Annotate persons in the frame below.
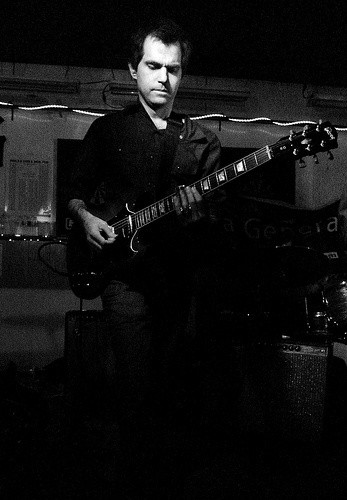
[52,12,232,472]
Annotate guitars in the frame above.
[66,118,339,300]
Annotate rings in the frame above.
[179,203,193,218]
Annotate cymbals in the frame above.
[263,242,330,288]
